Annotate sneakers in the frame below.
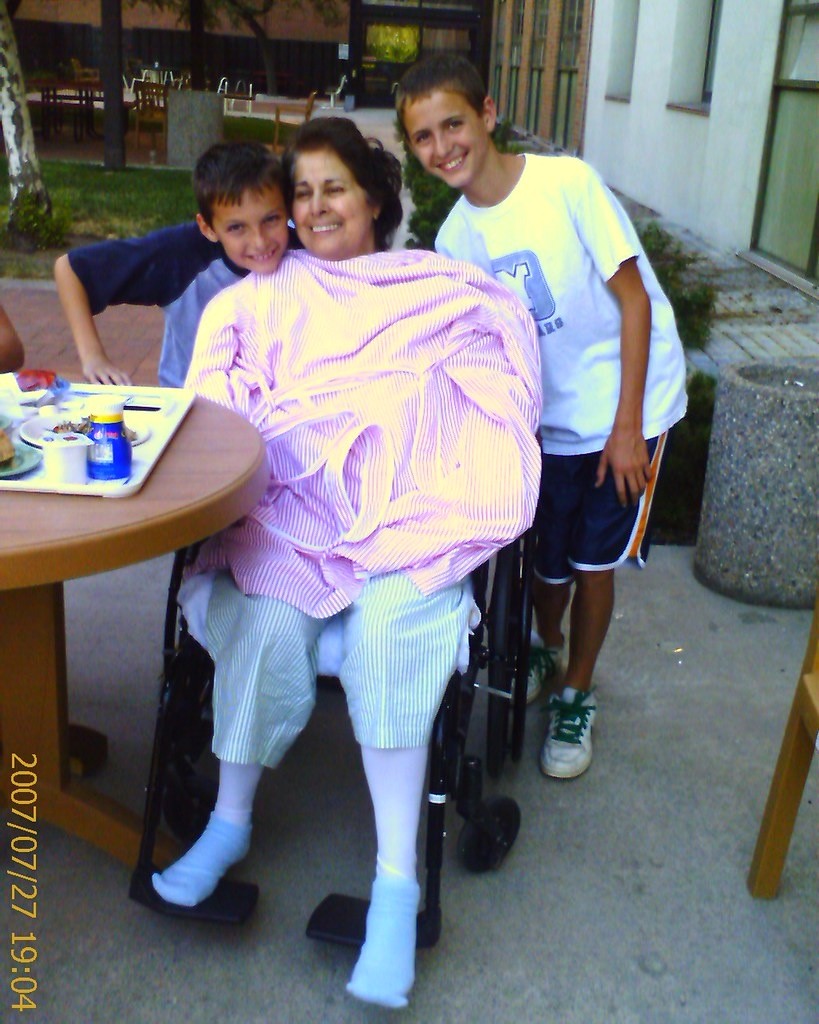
[511,628,565,703]
[541,685,599,779]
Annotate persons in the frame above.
[0,303,25,373]
[52,139,308,389]
[399,52,690,781]
[152,116,545,1008]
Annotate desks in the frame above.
[0,382,269,873]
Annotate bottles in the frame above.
[86,396,132,481]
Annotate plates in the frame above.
[19,410,152,457]
[14,374,71,395]
[0,444,43,480]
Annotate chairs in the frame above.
[271,88,319,154]
[323,74,348,109]
[24,68,261,158]
